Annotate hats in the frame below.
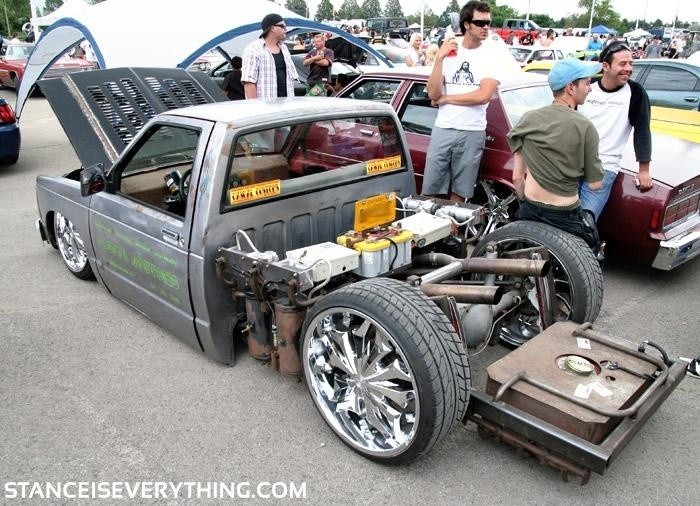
[548,56,603,92]
[258,13,283,38]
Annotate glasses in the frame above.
[466,19,491,28]
[274,24,287,29]
[603,41,627,62]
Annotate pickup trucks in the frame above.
[490,19,550,41]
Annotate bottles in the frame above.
[445,24,458,58]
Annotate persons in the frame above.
[402,28,700,67]
[222,13,365,148]
[71,43,85,60]
[506,60,600,276]
[575,41,654,221]
[420,0,498,233]
[450,61,473,84]
[88,44,95,63]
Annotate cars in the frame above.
[0,43,98,90]
[524,58,700,142]
[510,44,563,67]
[275,71,700,270]
[322,18,464,67]
[34,68,700,484]
[0,96,20,166]
